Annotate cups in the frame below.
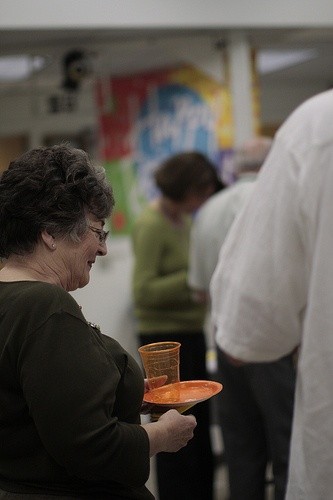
[137,342,181,403]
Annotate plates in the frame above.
[142,378,223,406]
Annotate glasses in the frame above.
[88,225,109,246]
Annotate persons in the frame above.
[211,89,333,500]
[0,144,197,500]
[187,137,297,500]
[132,150,228,500]
